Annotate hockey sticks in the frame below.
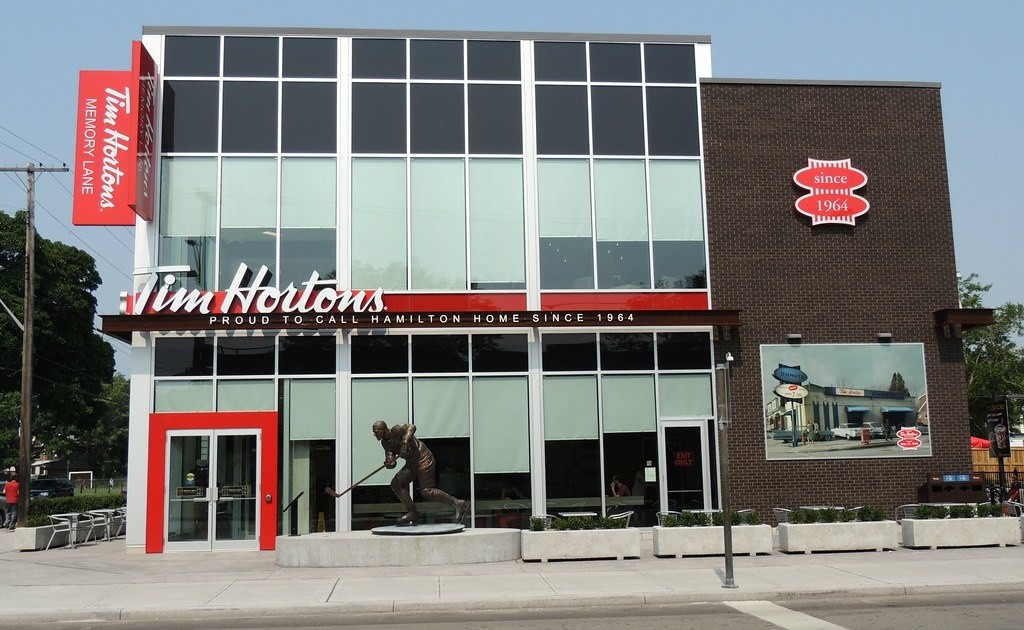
[325,448,412,498]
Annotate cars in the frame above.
[0,481,9,528]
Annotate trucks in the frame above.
[771,429,834,443]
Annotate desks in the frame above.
[558,511,598,530]
[923,501,978,519]
[800,506,845,522]
[683,509,724,526]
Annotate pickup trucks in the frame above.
[830,423,861,440]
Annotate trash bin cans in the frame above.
[917,473,957,503]
[954,473,986,503]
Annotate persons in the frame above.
[605,466,652,527]
[804,417,895,449]
[0,471,116,532]
[372,420,472,526]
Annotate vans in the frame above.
[862,421,887,439]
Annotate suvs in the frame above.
[29,476,75,501]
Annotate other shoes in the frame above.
[3,523,16,530]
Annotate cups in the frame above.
[994,424,1007,449]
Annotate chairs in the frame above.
[92,509,126,541]
[608,510,634,528]
[894,503,924,522]
[76,512,112,545]
[503,502,529,515]
[979,502,992,517]
[529,514,560,531]
[773,507,793,524]
[737,509,753,523]
[652,499,678,510]
[848,506,864,522]
[1002,500,1024,517]
[656,511,684,527]
[45,512,94,550]
